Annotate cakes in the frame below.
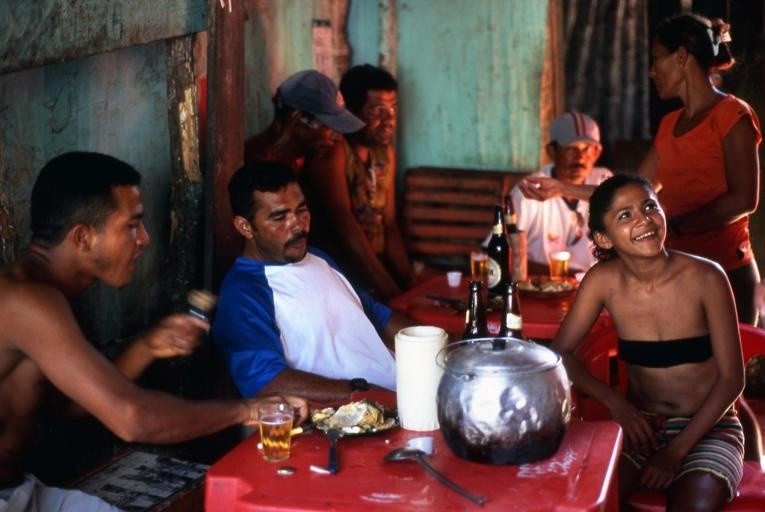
[328,404,385,430]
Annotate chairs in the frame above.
[620,319,765,512]
[402,167,505,261]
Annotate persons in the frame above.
[219,160,430,413]
[518,14,762,330]
[481,110,613,272]
[243,69,364,259]
[338,63,423,308]
[551,174,746,511]
[2,151,308,510]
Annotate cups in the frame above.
[471,250,489,312]
[258,403,293,461]
[551,253,568,281]
[448,272,462,287]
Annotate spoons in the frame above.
[383,448,486,503]
[325,428,345,473]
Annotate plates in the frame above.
[309,406,400,435]
[515,280,576,298]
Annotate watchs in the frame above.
[669,215,684,236]
[244,397,260,428]
[348,378,369,392]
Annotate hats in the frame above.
[273,70,367,135]
[548,110,602,151]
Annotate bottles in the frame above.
[488,209,508,304]
[464,282,489,340]
[497,284,522,340]
[504,208,526,281]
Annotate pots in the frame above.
[437,338,571,464]
